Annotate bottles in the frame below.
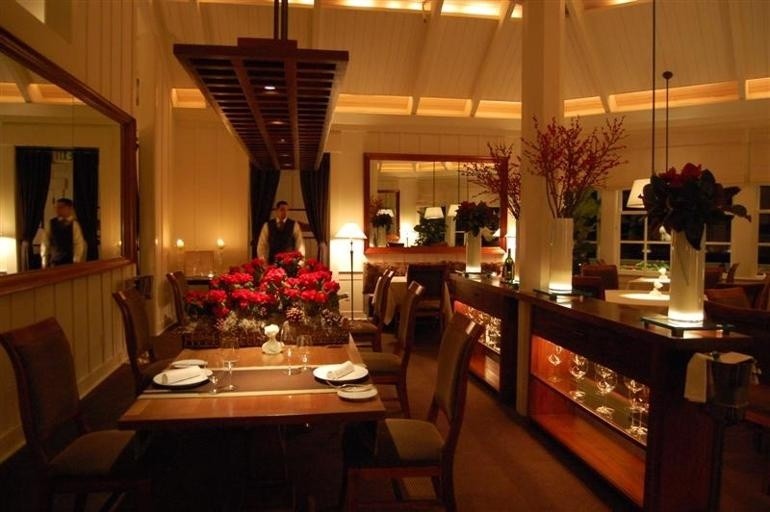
[504,248,513,281]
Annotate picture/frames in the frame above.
[184,248,214,278]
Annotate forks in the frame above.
[325,380,368,388]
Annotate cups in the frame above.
[623,375,644,435]
[593,365,617,415]
[297,334,313,374]
[204,354,225,395]
[568,352,589,398]
[281,326,301,376]
[629,389,649,437]
[221,337,240,392]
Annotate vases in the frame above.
[547,216,576,294]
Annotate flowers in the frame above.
[522,113,631,211]
[184,250,353,347]
[455,142,524,218]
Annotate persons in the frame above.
[257,201,305,269]
[40,198,85,268]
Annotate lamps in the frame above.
[627,3,655,208]
[447,162,462,218]
[425,161,444,223]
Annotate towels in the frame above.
[160,365,200,384]
[327,360,353,379]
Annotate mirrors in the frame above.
[361,151,508,254]
[0,25,139,291]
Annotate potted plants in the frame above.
[372,213,390,248]
[451,203,498,273]
[644,161,753,322]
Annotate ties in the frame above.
[279,221,283,228]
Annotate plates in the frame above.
[338,385,378,400]
[173,359,204,368]
[313,363,369,382]
[153,369,213,387]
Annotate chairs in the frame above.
[113,289,174,397]
[703,287,750,308]
[573,274,608,304]
[700,294,767,383]
[718,261,743,286]
[2,315,145,510]
[339,312,485,511]
[348,269,396,351]
[406,263,447,340]
[166,269,200,350]
[577,261,619,288]
[361,282,429,416]
[699,266,724,283]
